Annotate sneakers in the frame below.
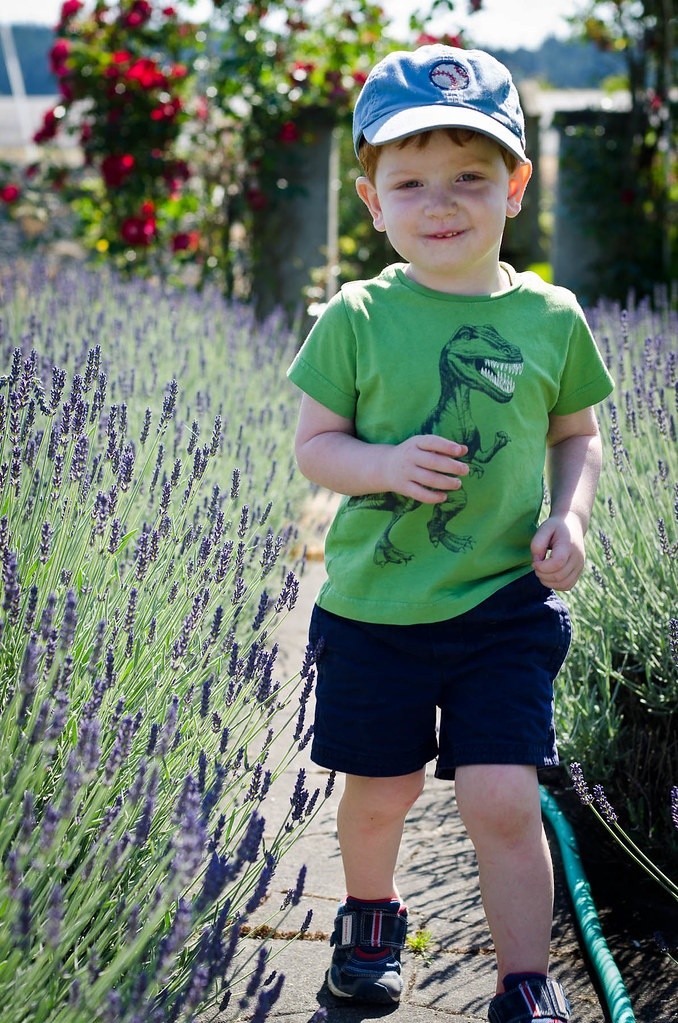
[327,893,408,1005]
[488,973,571,1023]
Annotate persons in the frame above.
[287,42,614,1023]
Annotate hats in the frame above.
[351,43,526,163]
